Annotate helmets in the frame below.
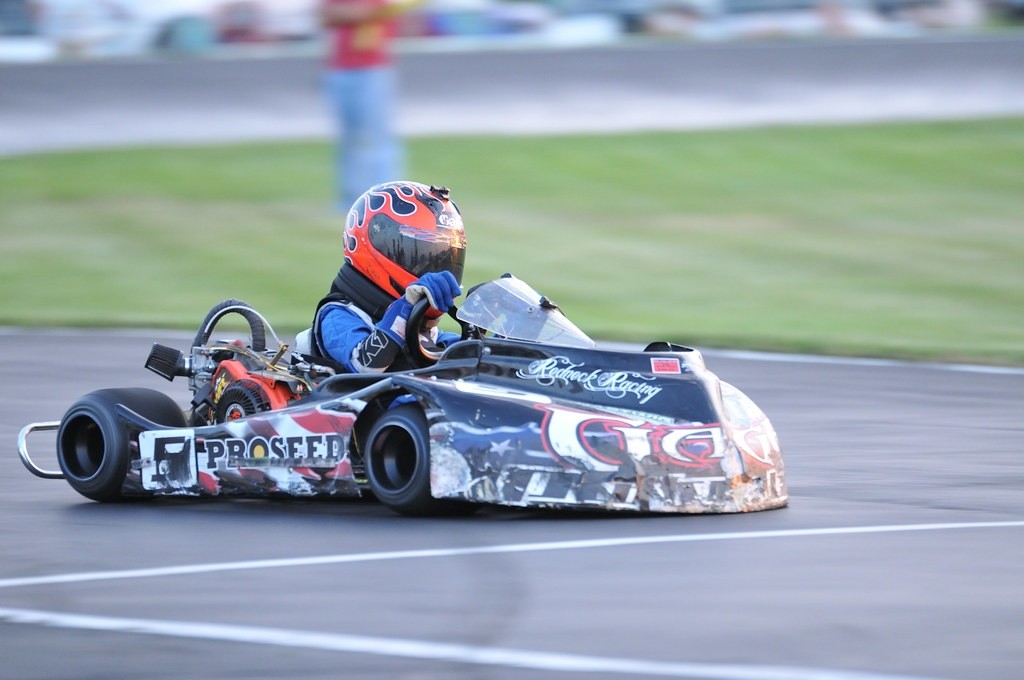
[343,180,467,318]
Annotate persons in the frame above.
[325,2,417,211]
[312,181,474,377]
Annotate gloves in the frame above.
[375,270,461,349]
